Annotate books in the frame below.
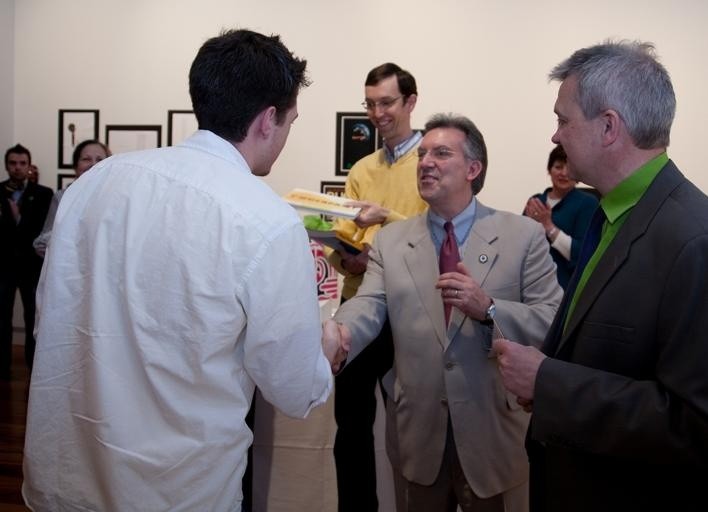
[283,187,363,221]
[308,228,364,256]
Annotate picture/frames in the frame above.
[57,109,199,190]
[320,111,384,222]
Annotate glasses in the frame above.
[361,95,405,109]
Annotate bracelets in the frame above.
[522,146,600,292]
[547,226,557,238]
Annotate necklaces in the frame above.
[548,193,559,204]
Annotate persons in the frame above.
[32,138,111,256]
[29,164,39,183]
[492,36,708,511]
[332,61,429,510]
[320,111,565,512]
[0,142,53,373]
[19,24,343,512]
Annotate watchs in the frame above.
[485,298,496,319]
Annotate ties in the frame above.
[540,204,606,356]
[440,222,460,330]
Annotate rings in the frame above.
[534,212,538,215]
[455,290,459,298]
